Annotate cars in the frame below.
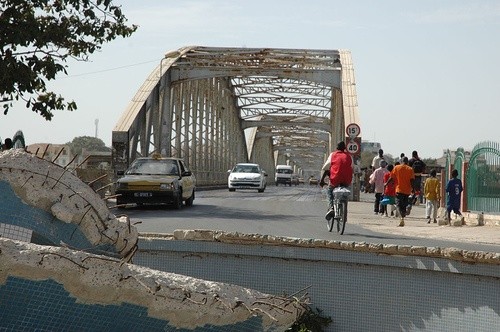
[227,163,268,193]
[114,148,197,209]
[291,171,318,186]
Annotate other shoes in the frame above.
[374,209,465,227]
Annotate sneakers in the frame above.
[324,209,333,220]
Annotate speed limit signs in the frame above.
[345,139,361,155]
[346,123,360,136]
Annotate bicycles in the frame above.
[324,181,352,235]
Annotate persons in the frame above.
[444,169,466,225]
[383,157,416,227]
[423,170,441,223]
[364,149,426,218]
[319,140,353,220]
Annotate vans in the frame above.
[274,165,293,187]
